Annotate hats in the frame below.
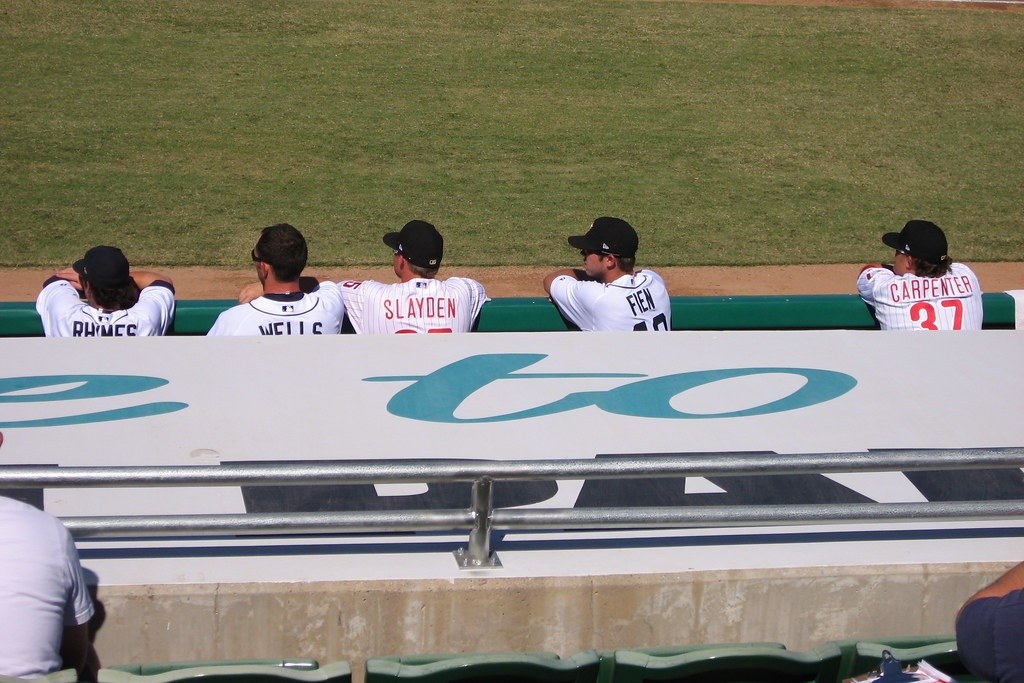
[382,220,444,269]
[567,216,639,257]
[72,245,129,283]
[882,220,947,263]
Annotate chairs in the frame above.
[3,637,983,683]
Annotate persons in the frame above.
[856,220,984,331]
[542,217,671,331]
[954,563,1024,683]
[208,224,344,334]
[0,491,97,681]
[338,221,492,333]
[36,246,177,337]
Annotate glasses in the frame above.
[251,248,272,265]
